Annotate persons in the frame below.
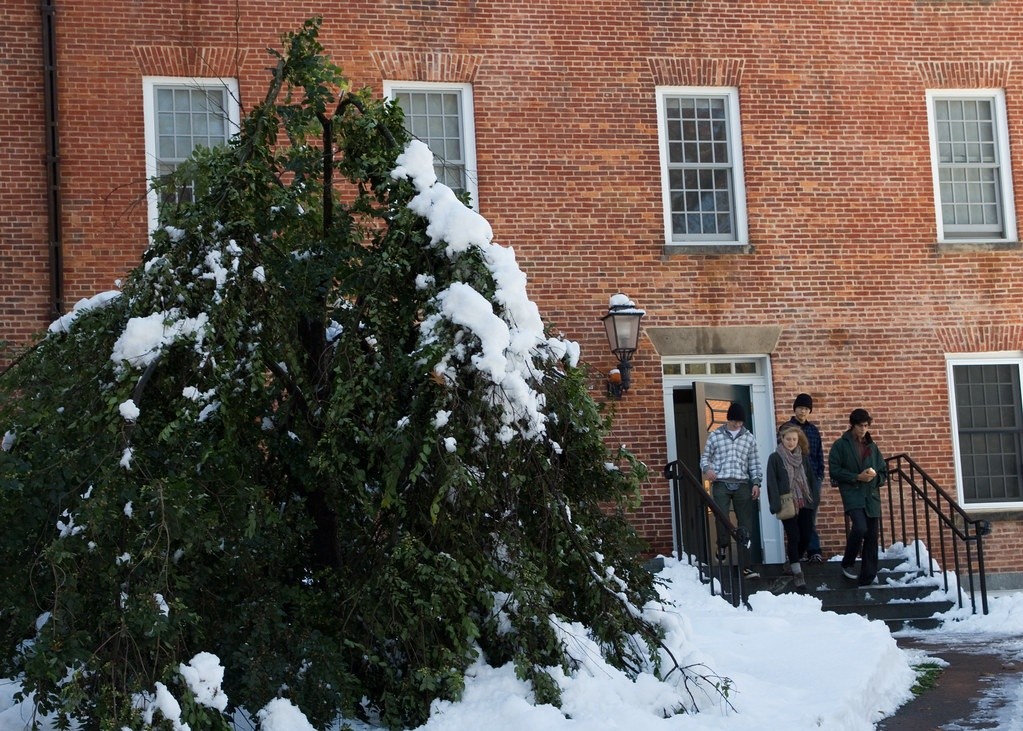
[777,393,825,563]
[768,426,814,587]
[700,403,763,579]
[828,408,888,586]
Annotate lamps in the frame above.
[599,293,645,399]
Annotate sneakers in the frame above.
[744,567,760,579]
[715,546,727,563]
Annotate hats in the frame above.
[727,403,745,421]
[793,394,812,413]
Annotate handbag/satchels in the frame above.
[775,493,795,519]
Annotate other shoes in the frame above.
[783,560,792,574]
[809,553,823,564]
[841,565,858,579]
[858,582,886,589]
[793,572,808,592]
[799,552,808,561]
